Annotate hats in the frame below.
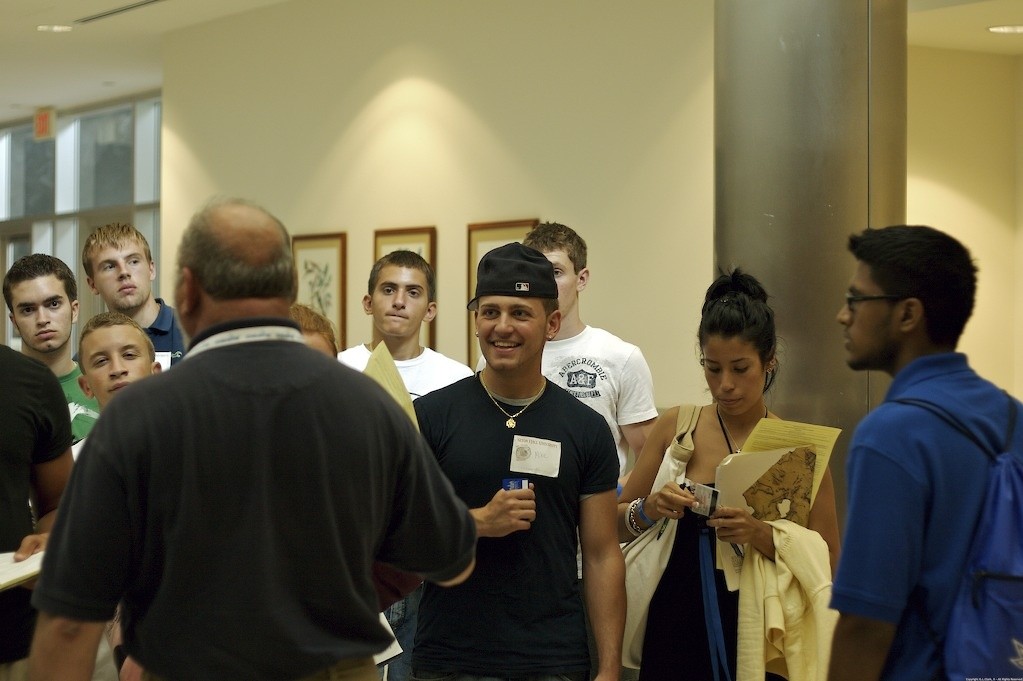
[465,242,559,313]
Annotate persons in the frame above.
[288,302,337,359]
[412,241,626,681]
[475,221,659,681]
[335,250,473,681]
[826,224,1023,681]
[613,270,839,681]
[23,204,478,681]
[0,224,185,681]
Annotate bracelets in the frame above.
[625,496,655,537]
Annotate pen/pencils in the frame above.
[657,482,686,540]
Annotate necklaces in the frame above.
[718,404,767,453]
[481,368,546,429]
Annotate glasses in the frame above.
[843,292,903,309]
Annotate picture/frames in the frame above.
[466,219,540,371]
[373,226,436,354]
[289,233,348,352]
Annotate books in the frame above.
[0,551,44,592]
